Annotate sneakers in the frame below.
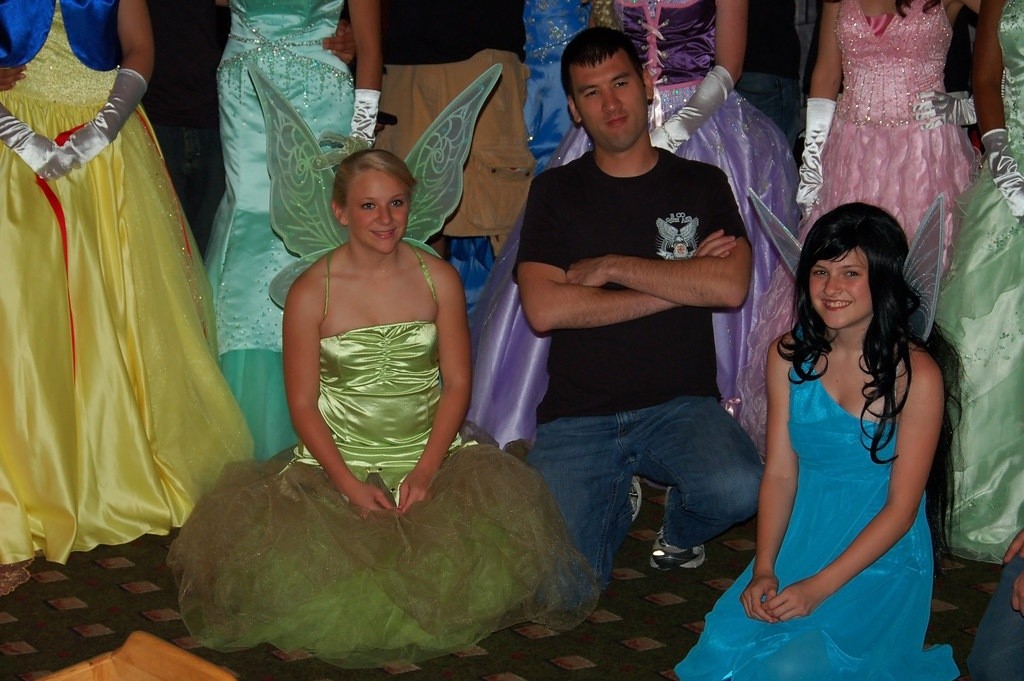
[629,473,642,522]
[649,485,706,570]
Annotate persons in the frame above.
[735,0,979,473]
[464,1,800,453]
[0,0,252,564]
[967,528,1024,680]
[170,145,597,669]
[908,0,1024,562]
[201,1,594,457]
[665,200,964,681]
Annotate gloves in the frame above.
[0,68,148,183]
[796,98,837,220]
[649,64,735,156]
[981,127,1024,226]
[349,89,382,150]
[912,90,978,132]
